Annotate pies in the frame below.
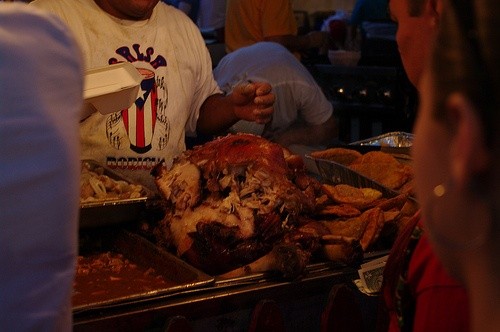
[317,183,408,252]
[312,148,406,189]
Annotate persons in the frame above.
[390,0,500,332]
[330,0,401,69]
[26,0,275,186]
[177,0,331,60]
[0,0,87,332]
[213,42,337,159]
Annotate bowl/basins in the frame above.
[328,50,362,66]
[82,62,146,116]
